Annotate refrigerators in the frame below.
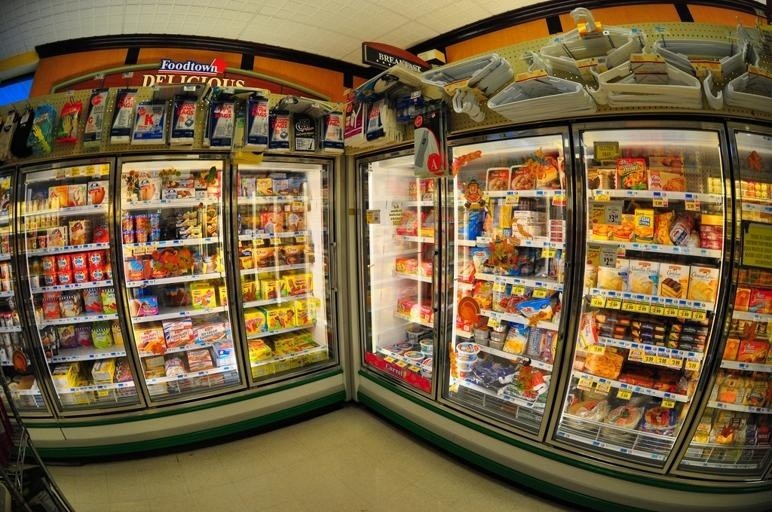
[0,149,347,462]
[345,133,771,512]
